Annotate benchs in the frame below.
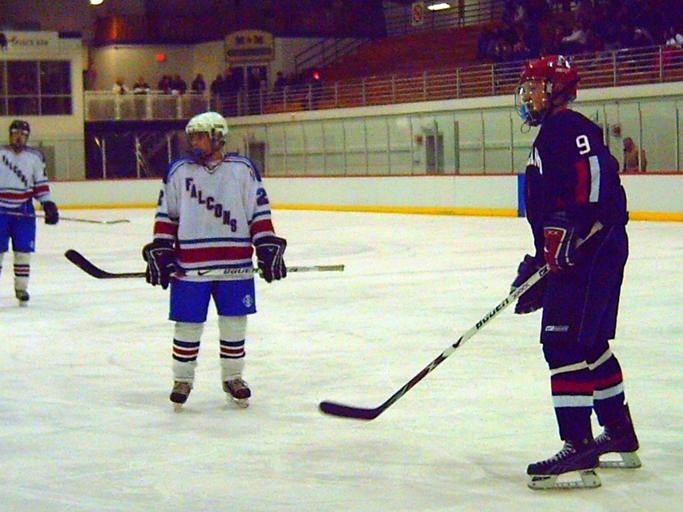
[263,0,683,112]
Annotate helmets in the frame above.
[516,55,579,127]
[185,112,228,158]
[9,120,30,137]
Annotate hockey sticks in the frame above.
[63,249,344,279]
[319,224,603,421]
[0,210,130,225]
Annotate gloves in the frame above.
[142,244,183,290]
[513,255,548,315]
[254,235,288,283]
[542,217,580,273]
[43,201,59,225]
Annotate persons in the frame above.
[0,119,59,302]
[509,52,641,477]
[113,65,322,117]
[622,137,646,172]
[475,1,683,93]
[144,110,287,402]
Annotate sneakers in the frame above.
[170,381,190,404]
[595,410,639,455]
[16,291,29,301]
[527,434,600,476]
[223,379,251,399]
